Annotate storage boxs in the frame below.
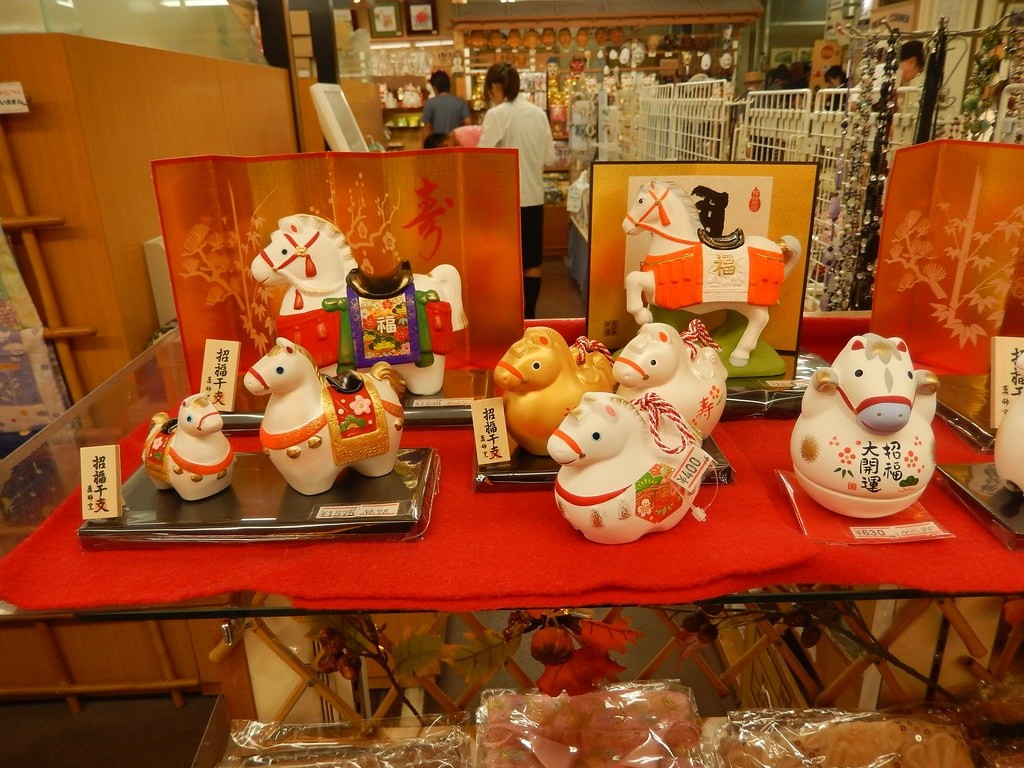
[150,332,192,409]
[143,232,179,329]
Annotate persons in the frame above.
[424,125,481,149]
[849,40,929,112]
[475,62,556,320]
[825,66,847,111]
[420,71,472,150]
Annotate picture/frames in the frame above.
[405,0,437,36]
[369,0,402,38]
